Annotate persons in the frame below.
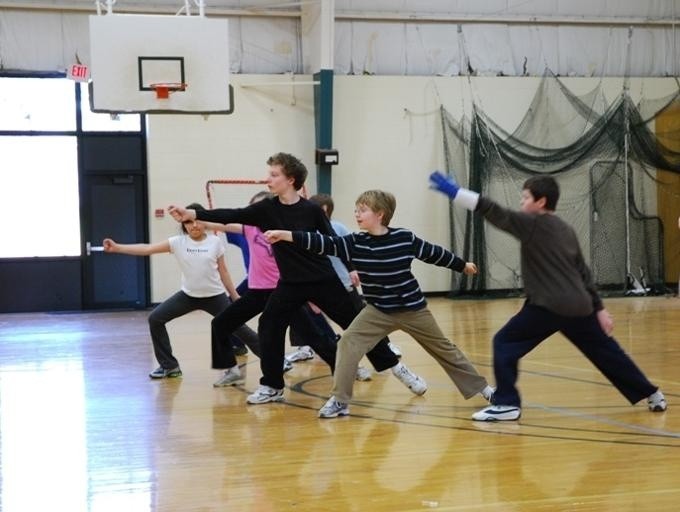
[264,188,497,419]
[168,152,428,406]
[427,169,668,422]
[102,204,293,378]
[287,193,407,362]
[193,190,373,386]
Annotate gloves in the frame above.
[427,170,479,212]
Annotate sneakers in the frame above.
[213,373,245,387]
[388,343,402,359]
[150,366,182,378]
[648,393,667,411]
[246,385,285,404]
[391,365,428,396]
[318,396,349,418]
[471,405,521,421]
[283,345,315,373]
[233,345,248,356]
[356,364,372,381]
